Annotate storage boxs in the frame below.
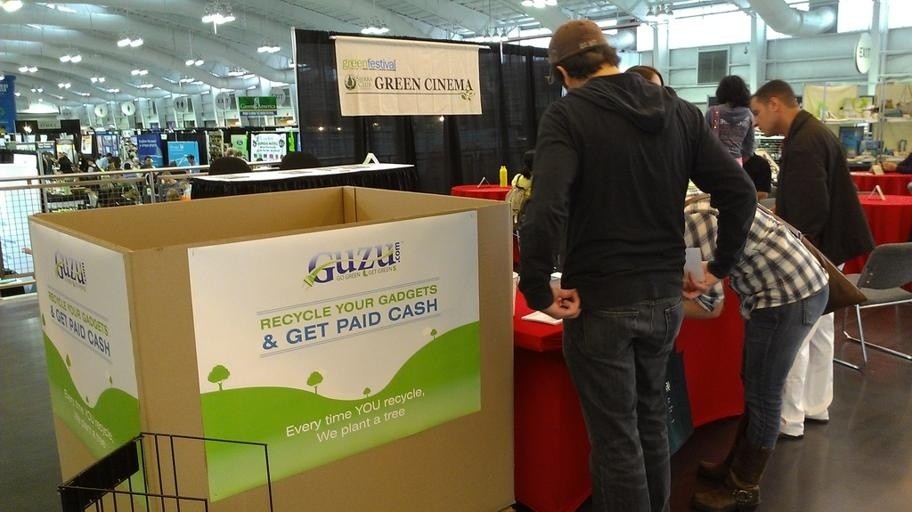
[24,182,530,511]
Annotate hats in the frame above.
[546,18,608,85]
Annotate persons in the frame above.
[881,152,912,173]
[40,149,200,194]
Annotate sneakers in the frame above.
[692,459,762,510]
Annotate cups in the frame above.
[512,270,521,319]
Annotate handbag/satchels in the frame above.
[801,233,868,317]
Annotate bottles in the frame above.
[499,165,508,188]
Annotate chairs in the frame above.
[831,238,912,378]
[758,198,777,210]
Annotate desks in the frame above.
[443,176,525,265]
[505,264,769,512]
[187,157,411,188]
[848,169,911,197]
[840,192,912,302]
